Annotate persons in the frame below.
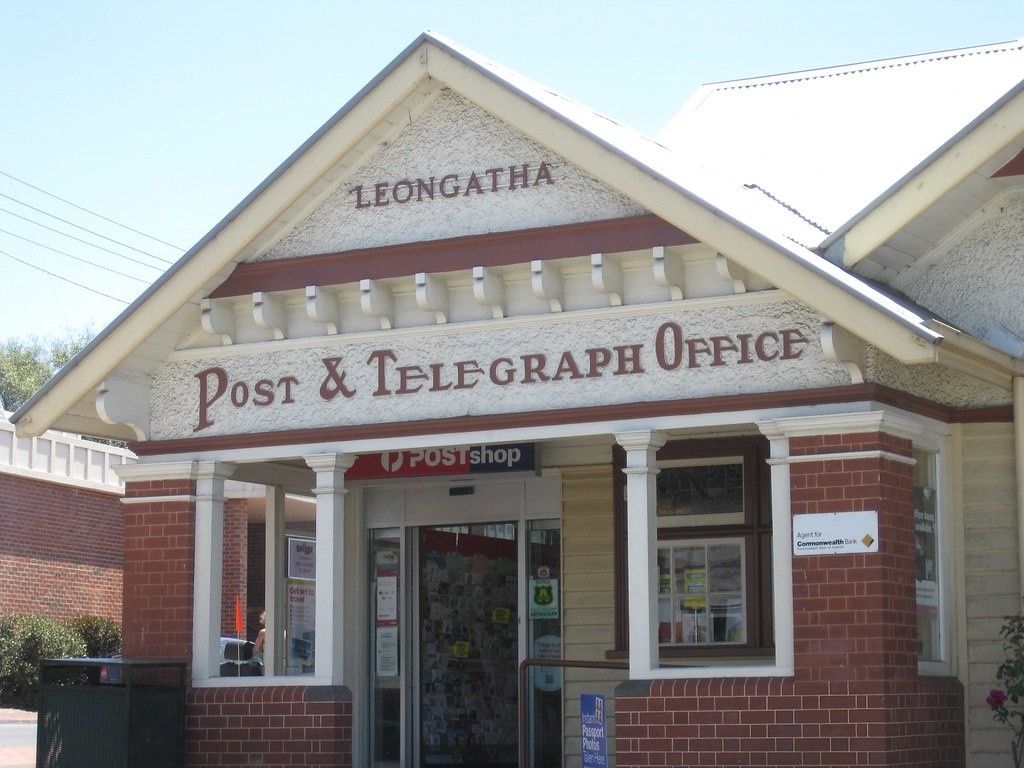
[254,612,287,676]
